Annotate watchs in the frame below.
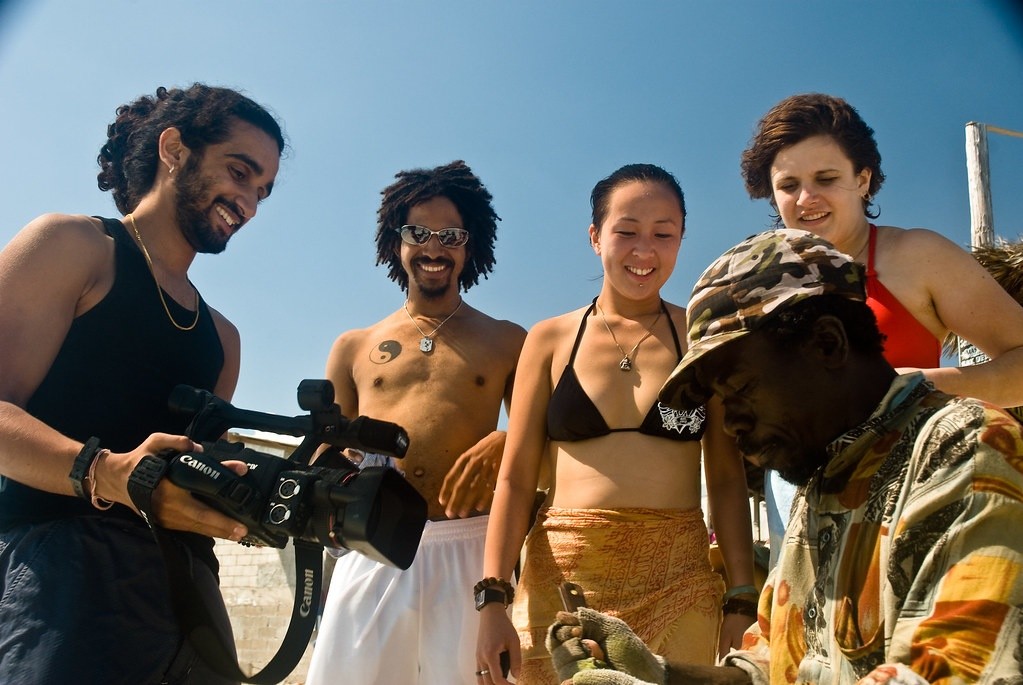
[475,587,509,611]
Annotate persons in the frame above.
[474,164,759,685]
[544,226,1023,685]
[739,91,1023,577]
[311,156,555,685]
[0,78,284,685]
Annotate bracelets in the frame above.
[68,435,115,511]
[721,597,758,620]
[473,576,515,604]
[721,584,760,604]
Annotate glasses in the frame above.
[394,224,475,249]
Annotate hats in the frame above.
[658,227,866,411]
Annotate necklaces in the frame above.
[123,213,200,331]
[594,299,664,371]
[403,293,464,353]
[852,236,870,260]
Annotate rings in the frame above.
[481,668,490,675]
[475,669,482,675]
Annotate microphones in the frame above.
[335,416,410,460]
